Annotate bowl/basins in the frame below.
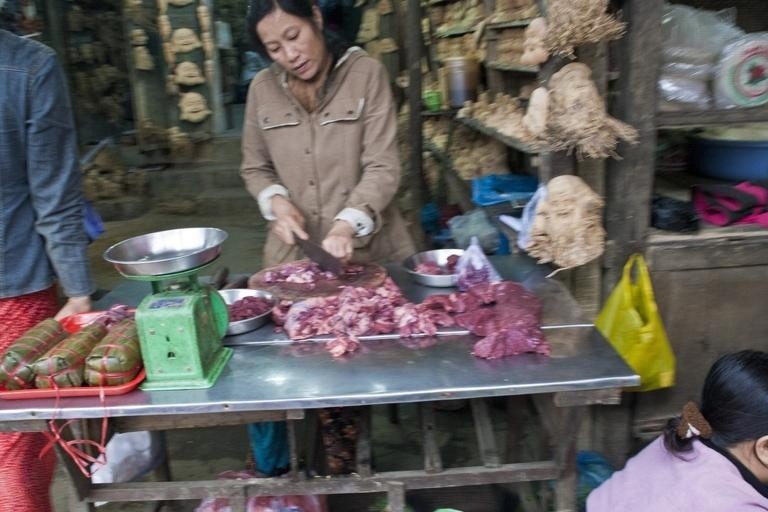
[690,132,768,182]
[216,288,278,336]
[400,248,466,287]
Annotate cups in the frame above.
[437,55,481,109]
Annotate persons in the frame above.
[578,348,767,512]
[237,0,417,275]
[0,27,96,510]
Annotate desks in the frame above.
[0,250,644,510]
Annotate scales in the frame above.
[102,228,235,393]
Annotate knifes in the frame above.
[293,233,346,279]
